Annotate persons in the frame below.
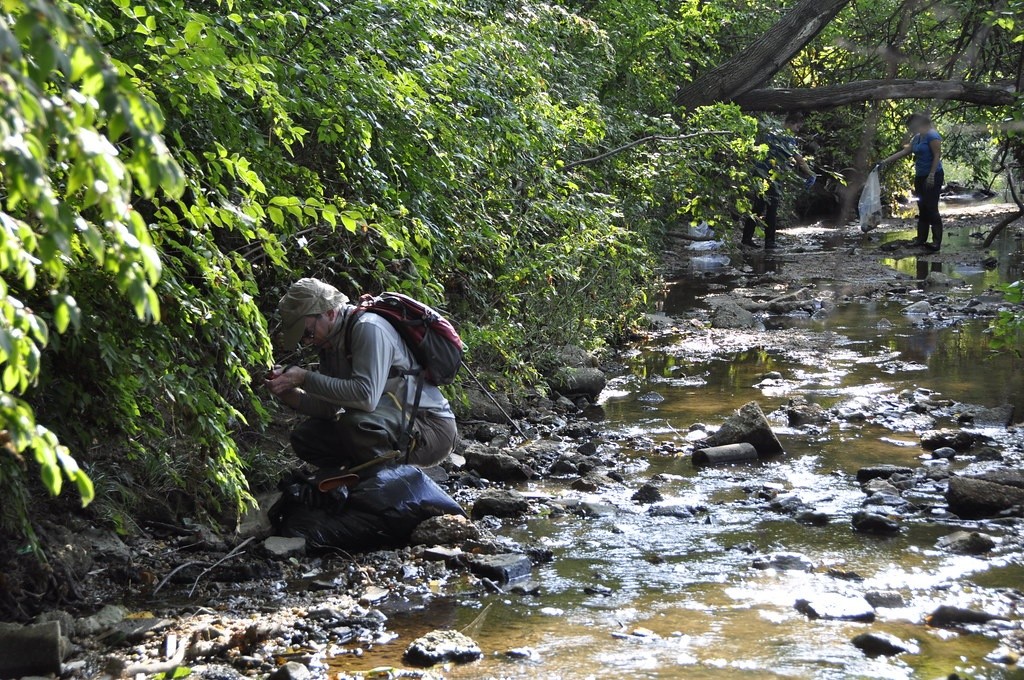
[264,277,458,480]
[741,111,816,249]
[877,113,944,252]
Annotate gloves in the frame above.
[925,172,934,189]
[805,177,815,189]
[877,159,890,171]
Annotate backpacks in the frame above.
[344,290,469,386]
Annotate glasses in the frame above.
[304,316,318,339]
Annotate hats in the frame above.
[284,317,303,349]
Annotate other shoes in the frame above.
[925,242,941,252]
[907,238,924,245]
[765,243,778,249]
[742,238,762,248]
